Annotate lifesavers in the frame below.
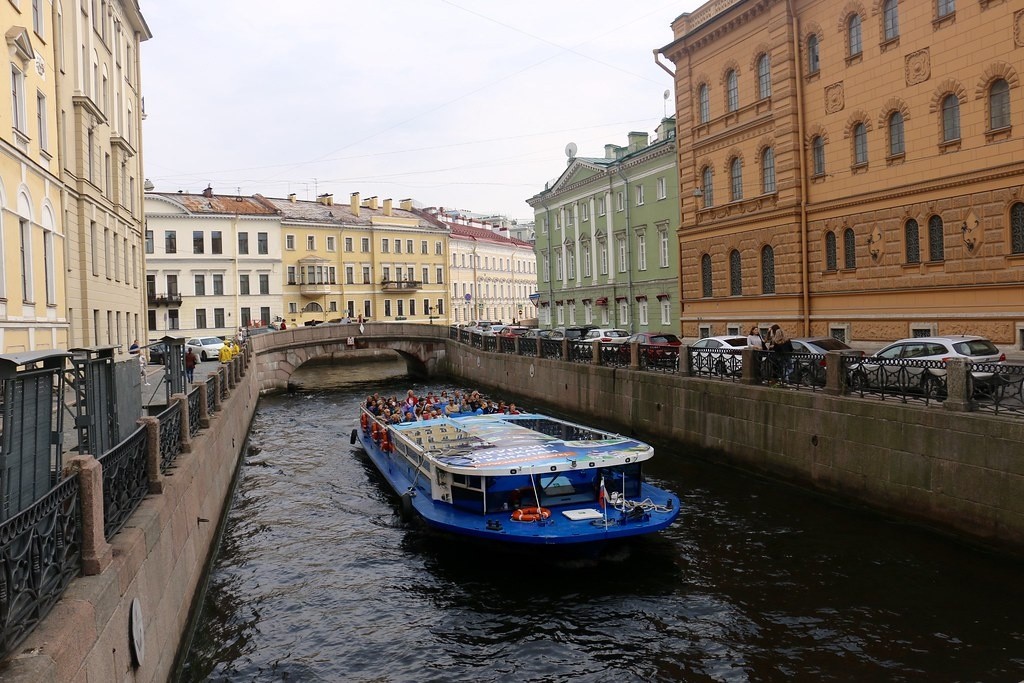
[400,494,412,521]
[361,413,367,433]
[380,428,387,451]
[513,507,551,521]
[371,422,377,443]
[350,429,357,445]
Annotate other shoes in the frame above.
[191,381,193,383]
[145,383,151,386]
[188,379,190,382]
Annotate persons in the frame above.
[911,347,919,354]
[748,326,764,381]
[186,347,195,384]
[219,340,232,364]
[138,350,152,386]
[129,340,140,353]
[765,324,780,385]
[231,341,239,355]
[234,313,363,344]
[774,330,795,390]
[484,320,557,336]
[367,390,522,424]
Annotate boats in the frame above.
[351,396,683,547]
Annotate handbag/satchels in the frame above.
[762,341,767,350]
[774,340,794,353]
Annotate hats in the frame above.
[223,340,229,344]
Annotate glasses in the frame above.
[397,413,399,414]
[449,400,454,401]
[424,413,428,414]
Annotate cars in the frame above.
[573,328,633,353]
[846,334,1012,401]
[150,344,170,365]
[763,336,866,385]
[521,329,552,339]
[452,319,505,337]
[185,337,234,362]
[544,326,590,346]
[675,335,750,375]
[494,326,530,339]
[619,331,682,359]
